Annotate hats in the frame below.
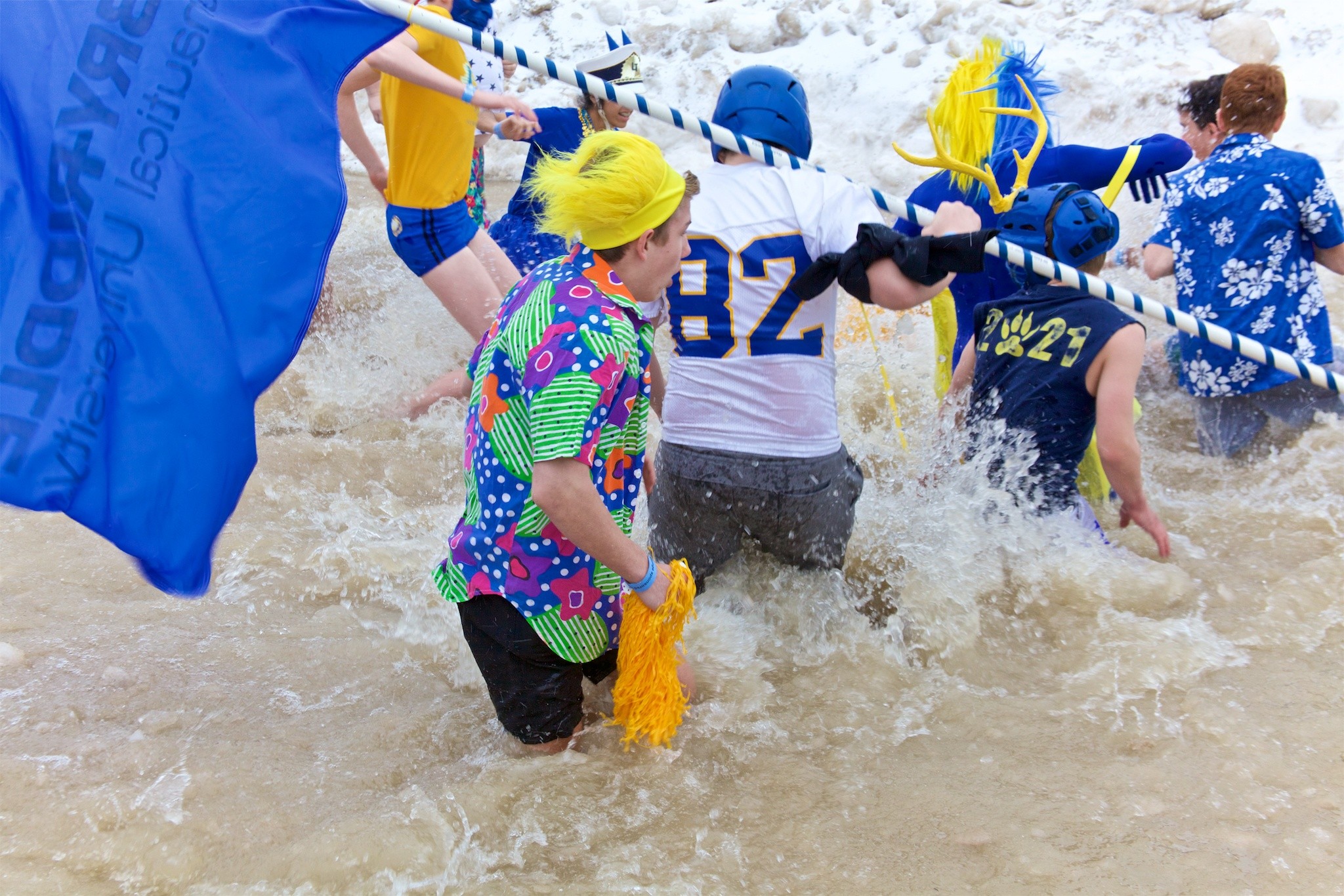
[996,181,1119,284]
[576,43,647,95]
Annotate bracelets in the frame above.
[625,556,657,592]
[460,84,475,103]
[494,122,508,141]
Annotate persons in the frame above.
[365,9,518,233]
[1178,74,1235,163]
[427,128,692,748]
[1138,61,1344,457]
[627,63,981,596]
[941,181,1170,558]
[475,44,646,280]
[335,0,541,424]
[889,37,1196,504]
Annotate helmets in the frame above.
[710,64,813,161]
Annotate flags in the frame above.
[0,0,414,599]
